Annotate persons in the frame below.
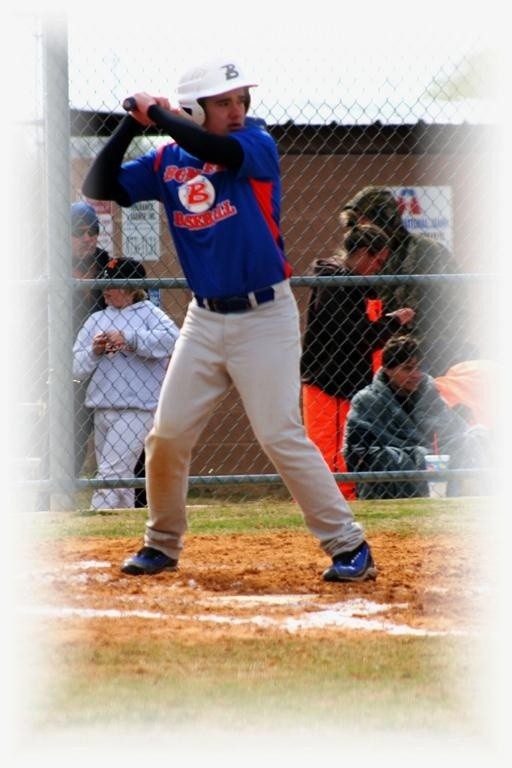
[81,59,377,582]
[341,342,467,498]
[71,202,180,509]
[302,224,416,497]
[343,186,463,378]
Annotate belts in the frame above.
[193,286,274,313]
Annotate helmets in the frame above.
[176,57,258,126]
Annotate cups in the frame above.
[426,453,450,498]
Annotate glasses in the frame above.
[72,226,97,238]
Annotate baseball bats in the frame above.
[123,97,267,129]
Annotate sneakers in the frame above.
[121,547,177,575]
[321,540,376,581]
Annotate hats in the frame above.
[104,257,146,279]
[71,202,99,228]
